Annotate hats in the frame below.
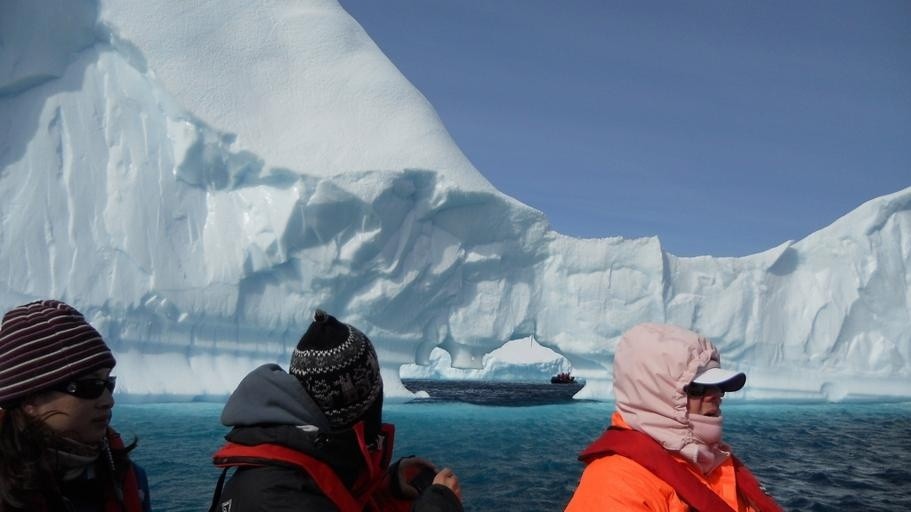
[289,310,382,427]
[1,299,115,404]
[693,358,746,391]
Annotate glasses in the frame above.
[56,377,115,399]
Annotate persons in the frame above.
[0,299,150,512]
[206,308,464,511]
[562,320,789,512]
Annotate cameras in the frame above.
[410,466,438,495]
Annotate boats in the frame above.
[400,377,586,405]
